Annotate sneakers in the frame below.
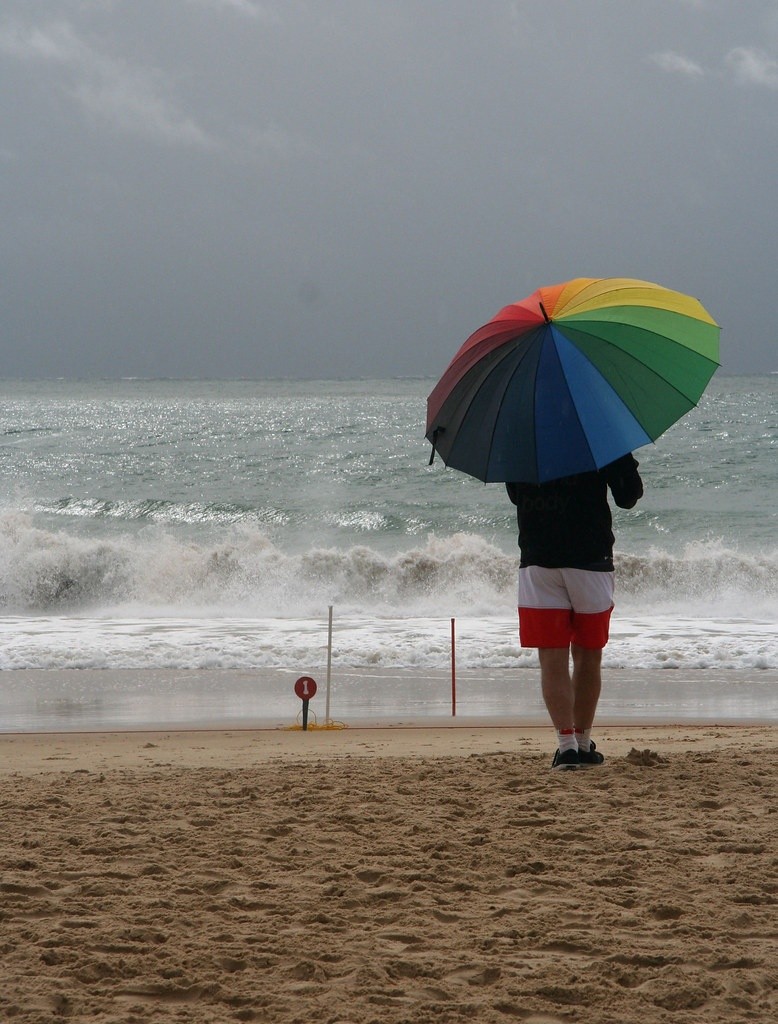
[550,748,582,770]
[578,738,604,767]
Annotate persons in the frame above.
[505,452,643,770]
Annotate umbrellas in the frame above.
[423,277,723,487]
